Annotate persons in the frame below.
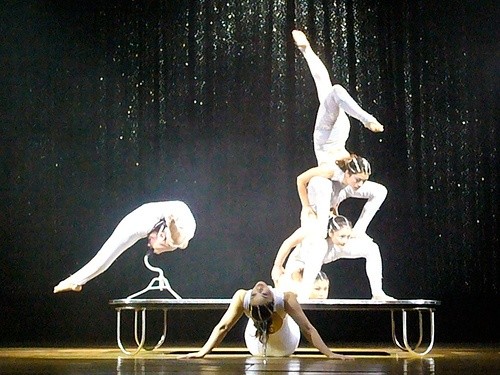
[292,29,384,219]
[179,281,356,360]
[271,177,397,300]
[54,200,197,293]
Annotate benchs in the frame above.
[109,299,441,356]
